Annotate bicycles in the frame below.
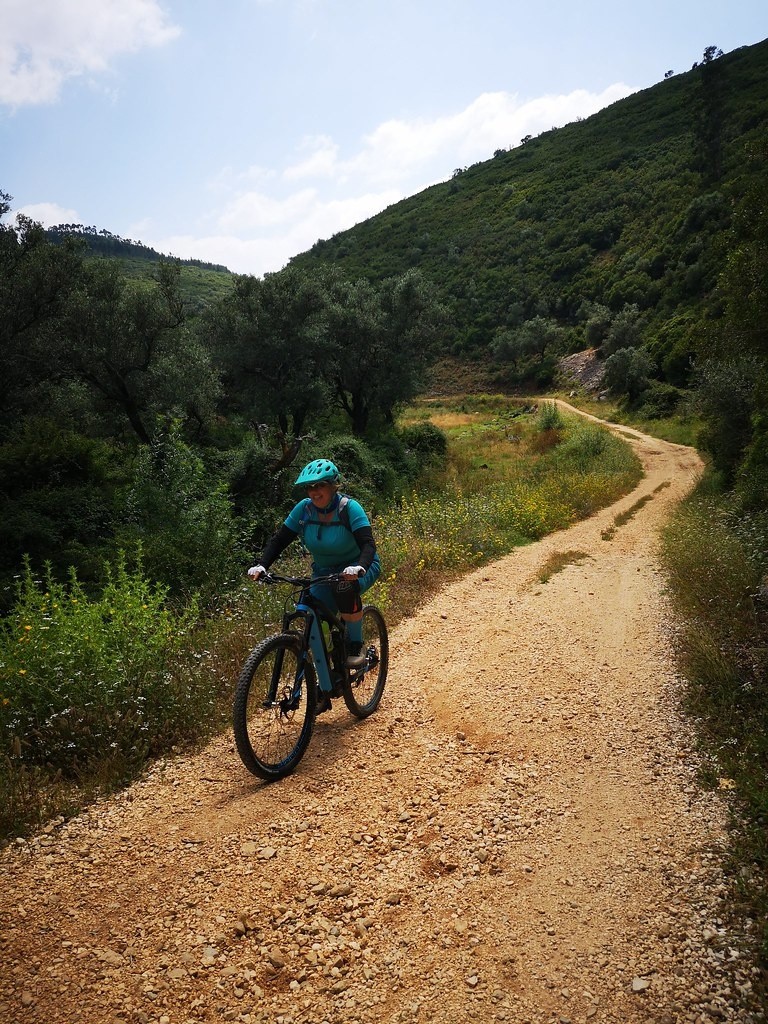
[229,571,391,782]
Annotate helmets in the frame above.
[295,459,338,485]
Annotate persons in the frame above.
[247,459,382,715]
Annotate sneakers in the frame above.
[347,641,367,666]
[315,686,324,714]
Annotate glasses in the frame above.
[303,481,329,492]
